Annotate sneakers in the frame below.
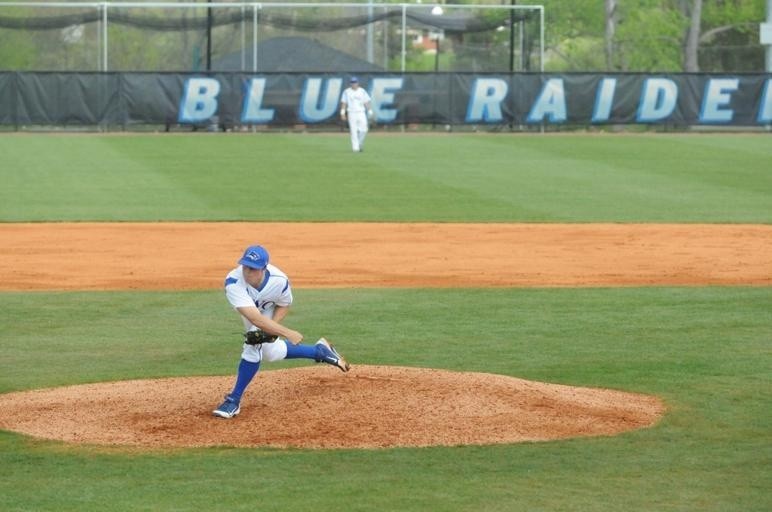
[211,394,240,418]
[314,338,351,371]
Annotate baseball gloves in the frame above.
[244,329,278,344]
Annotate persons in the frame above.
[411,29,446,55]
[339,76,374,153]
[210,245,350,420]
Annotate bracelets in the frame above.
[368,108,373,115]
[341,109,347,114]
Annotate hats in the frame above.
[238,246,269,269]
[350,77,358,82]
